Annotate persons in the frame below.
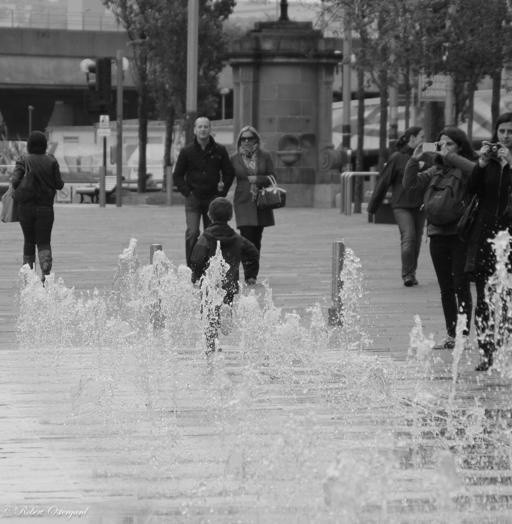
[173,114,236,277]
[461,112,512,371]
[216,124,278,292]
[188,196,261,361]
[364,124,433,286]
[401,126,479,350]
[10,128,66,289]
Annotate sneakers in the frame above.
[476,358,493,371]
[402,274,418,287]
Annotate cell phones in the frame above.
[422,142,438,153]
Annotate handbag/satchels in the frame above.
[15,155,54,207]
[256,187,286,209]
[0,186,16,224]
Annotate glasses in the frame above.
[239,137,256,141]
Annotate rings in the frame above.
[503,148,503,150]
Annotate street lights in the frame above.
[79,55,129,175]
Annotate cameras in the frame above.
[488,142,500,154]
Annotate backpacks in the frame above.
[424,154,479,225]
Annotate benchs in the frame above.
[76,175,124,203]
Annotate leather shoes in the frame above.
[444,337,455,350]
